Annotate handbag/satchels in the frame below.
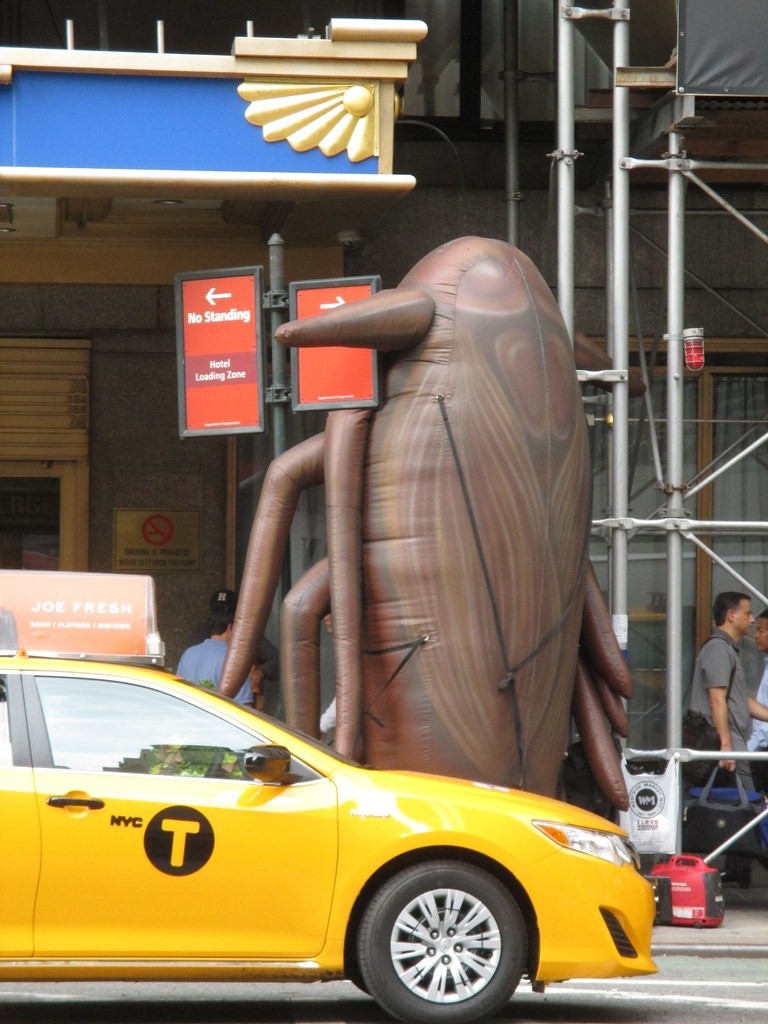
[682,765,768,858]
[658,636,736,786]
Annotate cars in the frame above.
[1,570,661,1024]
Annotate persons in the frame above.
[686,590,768,889]
[174,586,339,734]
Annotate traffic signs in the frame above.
[175,263,269,439]
[289,274,385,413]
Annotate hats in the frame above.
[210,590,237,611]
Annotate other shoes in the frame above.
[719,865,751,887]
